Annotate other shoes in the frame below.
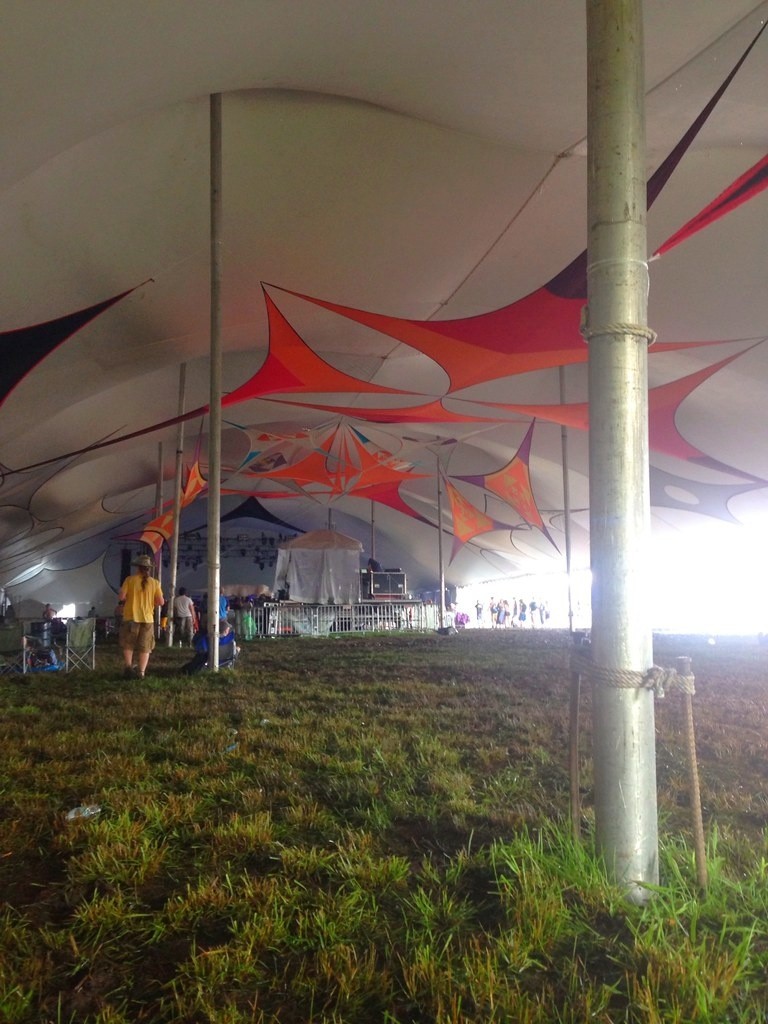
[138,673,145,680]
[123,667,134,679]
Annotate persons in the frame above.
[367,557,382,573]
[115,553,165,680]
[3,603,100,648]
[164,584,280,670]
[476,594,553,630]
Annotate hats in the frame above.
[219,620,233,632]
[130,556,153,566]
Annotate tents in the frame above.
[270,530,365,606]
